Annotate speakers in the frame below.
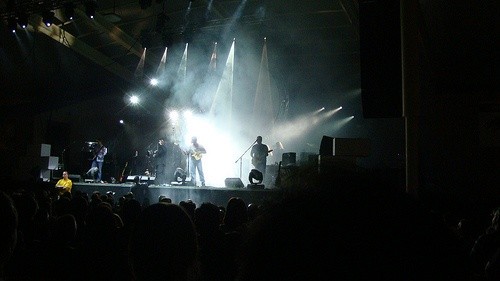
[68,175,83,182]
[225,178,244,188]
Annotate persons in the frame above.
[274,142,284,162]
[55,170,72,200]
[90,140,108,182]
[250,136,268,184]
[153,139,167,186]
[0,188,500,281]
[184,137,207,187]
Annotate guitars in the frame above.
[252,149,274,167]
[89,143,106,161]
[188,145,202,161]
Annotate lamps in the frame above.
[66,4,74,20]
[42,9,52,27]
[85,0,97,19]
[6,13,27,33]
